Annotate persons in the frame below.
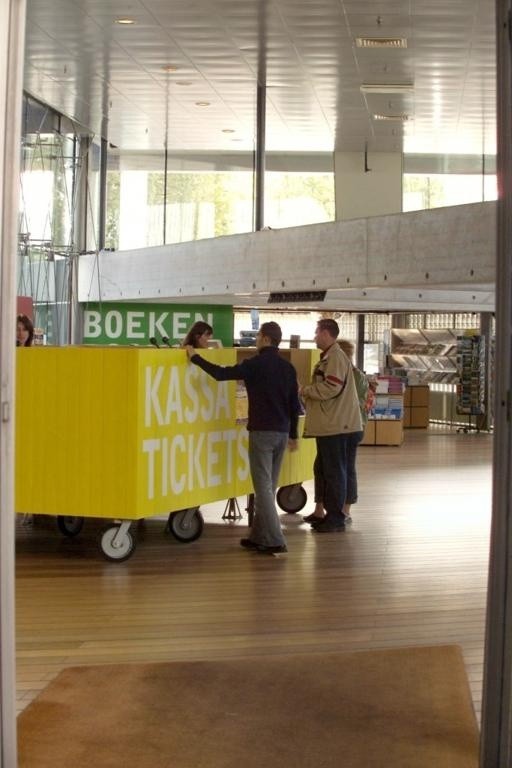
[181,320,222,348]
[304,338,368,525]
[296,319,364,532]
[16,313,33,347]
[186,320,298,554]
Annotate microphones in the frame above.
[150,336,160,350]
[162,336,172,348]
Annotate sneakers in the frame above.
[251,542,288,554]
[240,538,262,549]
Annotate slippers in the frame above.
[303,514,352,532]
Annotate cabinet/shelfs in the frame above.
[358,327,485,447]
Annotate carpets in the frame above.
[10,640,487,766]
